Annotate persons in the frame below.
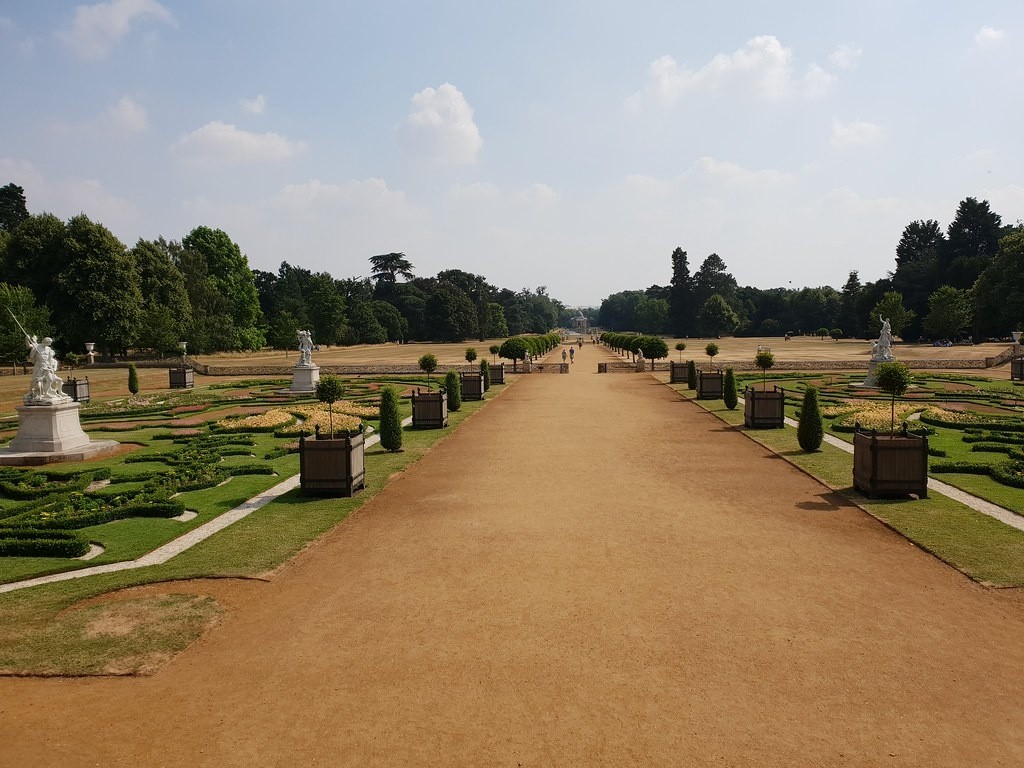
[315,344,320,351]
[638,348,643,359]
[579,341,582,348]
[758,345,762,352]
[569,347,575,363]
[562,349,567,362]
[934,339,952,346]
[871,315,892,359]
[296,330,316,367]
[593,339,599,345]
[766,344,770,352]
[525,350,530,359]
[25,335,67,399]
[919,336,922,344]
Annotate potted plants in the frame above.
[487,344,506,385]
[853,360,928,502]
[299,373,366,498]
[62,351,90,403]
[412,352,448,431]
[169,345,194,388]
[744,348,785,429]
[669,342,691,383]
[696,343,723,400]
[460,347,484,401]
